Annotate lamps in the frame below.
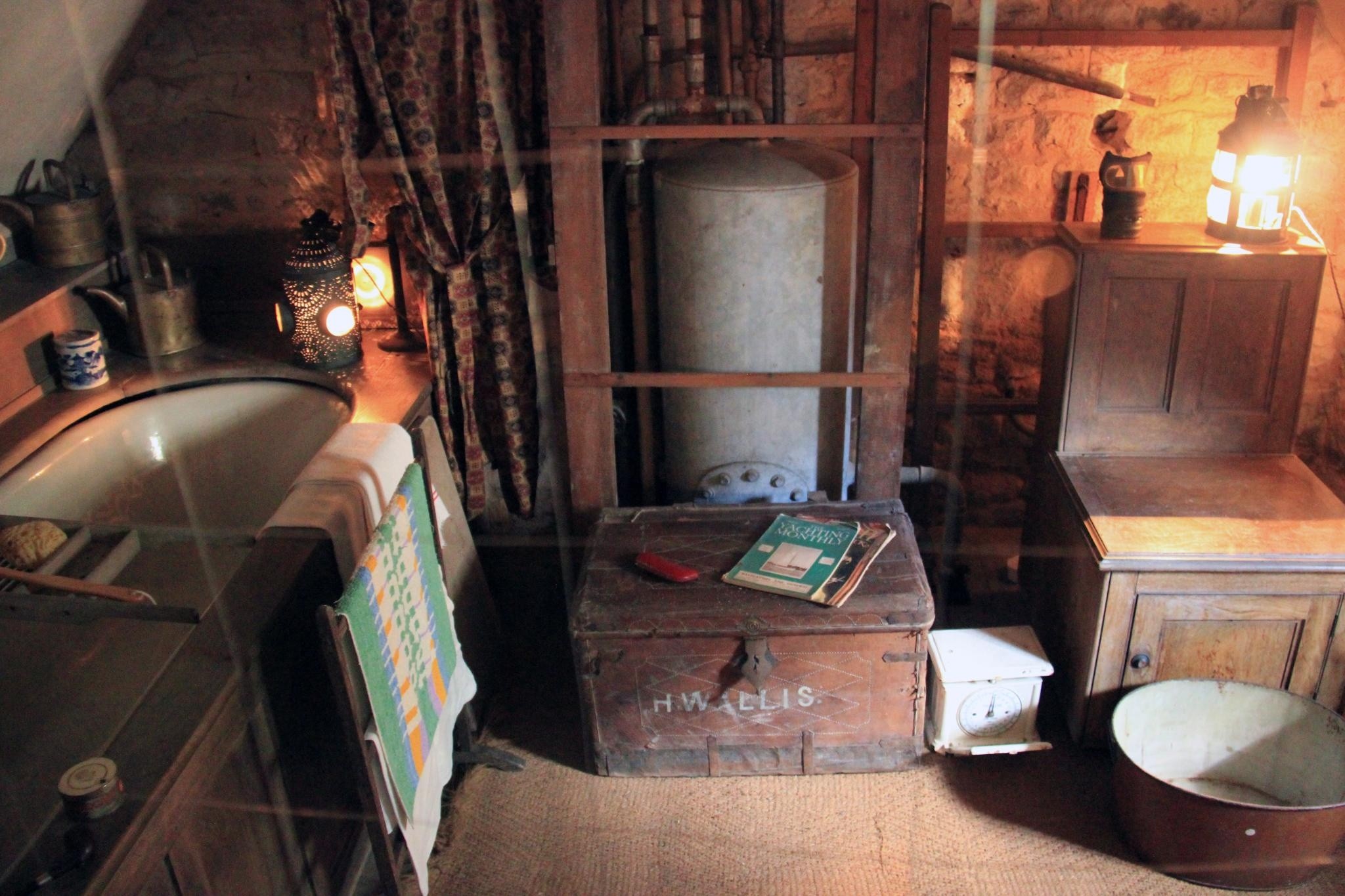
[273,206,365,372]
[1205,84,1305,244]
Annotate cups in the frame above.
[52,330,110,392]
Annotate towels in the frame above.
[334,465,481,818]
[282,417,457,557]
[354,635,481,896]
[410,415,479,604]
[254,479,368,575]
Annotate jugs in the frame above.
[88,244,206,357]
[0,157,107,267]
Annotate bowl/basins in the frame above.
[1107,676,1344,891]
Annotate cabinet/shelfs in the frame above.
[1016,222,1344,747]
[103,535,370,896]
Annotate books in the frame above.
[721,513,861,596]
[788,511,897,606]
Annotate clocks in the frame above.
[923,624,1055,758]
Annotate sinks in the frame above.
[0,368,360,530]
